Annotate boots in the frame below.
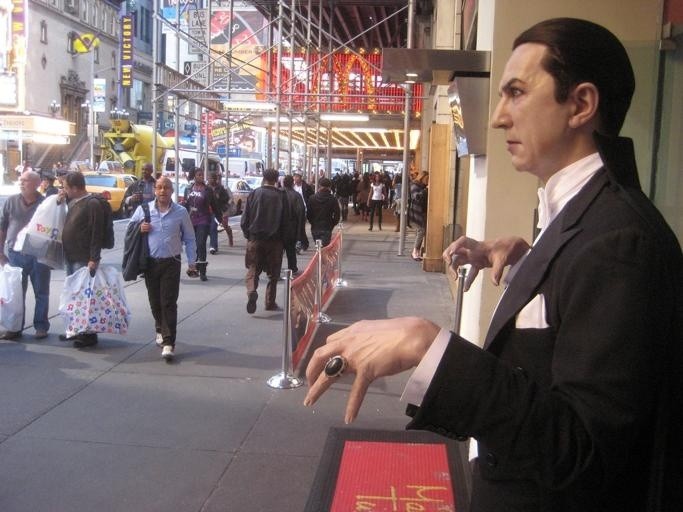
[395,217,400,232]
[197,262,208,281]
[226,226,234,246]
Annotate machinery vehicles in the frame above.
[96,107,165,175]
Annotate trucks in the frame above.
[159,148,224,204]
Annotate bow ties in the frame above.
[536,153,605,229]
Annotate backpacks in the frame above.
[85,195,114,249]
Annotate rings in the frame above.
[324,355,347,378]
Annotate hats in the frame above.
[294,170,302,176]
[42,171,56,180]
[56,169,68,176]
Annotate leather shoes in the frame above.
[0,332,22,340]
[411,254,423,261]
[36,330,47,339]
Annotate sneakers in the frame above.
[379,224,381,230]
[156,332,164,346]
[266,304,277,310]
[247,291,258,313]
[74,335,97,348]
[162,345,174,359]
[368,225,373,230]
[293,271,303,278]
[59,335,77,341]
[210,247,216,254]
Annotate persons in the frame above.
[330,169,419,232]
[122,162,233,281]
[52,162,62,174]
[411,170,429,260]
[127,178,195,359]
[227,170,262,178]
[303,18,683,512]
[95,161,99,170]
[240,168,340,313]
[22,160,28,172]
[0,169,105,346]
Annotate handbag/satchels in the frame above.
[367,193,373,208]
[13,193,68,269]
[0,264,23,332]
[57,266,130,339]
[222,186,237,217]
[207,203,215,216]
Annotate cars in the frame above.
[82,173,140,219]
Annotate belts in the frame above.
[159,255,181,262]
[8,249,27,256]
